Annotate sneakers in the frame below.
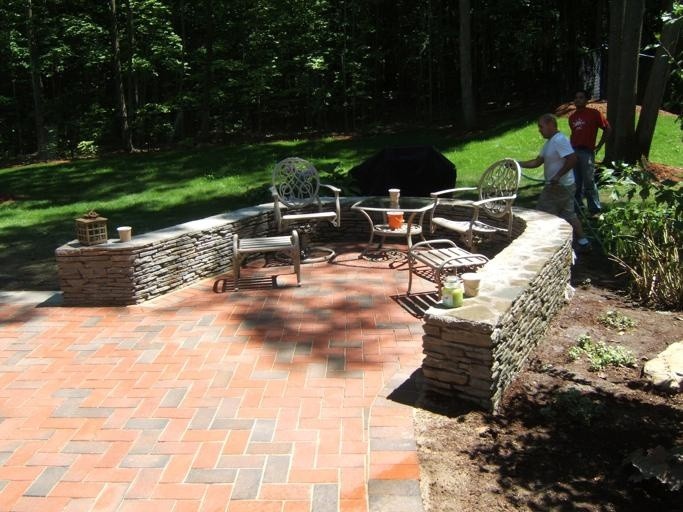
[573,240,593,254]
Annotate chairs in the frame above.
[267,156,342,264]
[429,160,522,249]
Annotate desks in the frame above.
[348,195,439,263]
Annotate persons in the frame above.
[502,109,594,256]
[566,88,613,221]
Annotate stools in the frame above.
[405,238,490,298]
[232,229,302,293]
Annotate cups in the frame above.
[388,188,401,201]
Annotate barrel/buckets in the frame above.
[387,212,405,229]
